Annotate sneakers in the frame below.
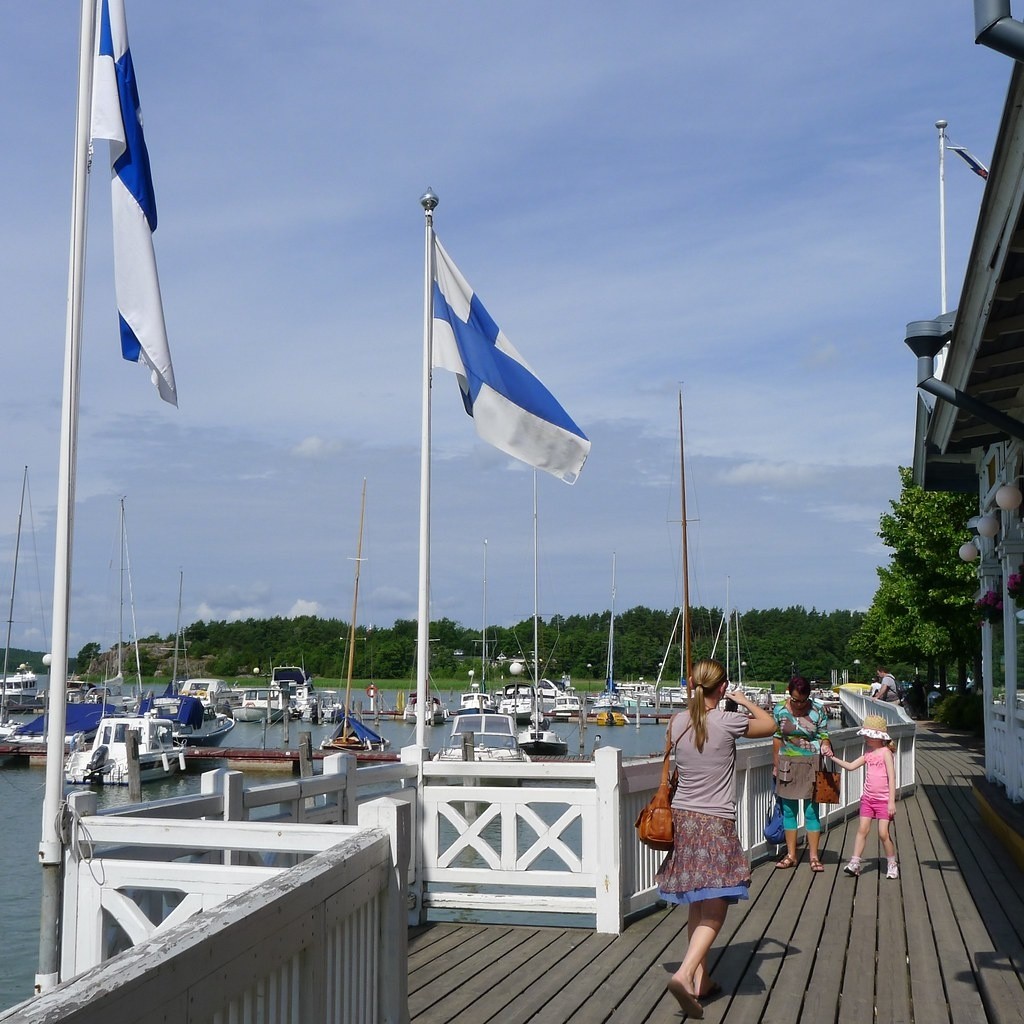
[843,862,861,876]
[886,862,899,879]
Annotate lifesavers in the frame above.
[367,687,377,698]
[196,690,207,698]
[245,702,255,707]
[179,691,189,696]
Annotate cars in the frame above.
[897,676,1024,711]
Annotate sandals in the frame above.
[809,857,824,871]
[775,855,798,868]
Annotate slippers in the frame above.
[666,979,704,1018]
[696,981,722,1000]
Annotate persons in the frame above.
[868,667,901,707]
[763,676,840,871]
[912,674,926,718]
[821,716,899,880]
[634,657,777,1018]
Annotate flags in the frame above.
[432,229,592,485]
[90,0,180,407]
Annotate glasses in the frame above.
[790,696,809,703]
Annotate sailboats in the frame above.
[63,492,187,786]
[0,384,844,765]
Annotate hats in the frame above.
[856,715,892,740]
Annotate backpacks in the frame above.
[886,673,905,694]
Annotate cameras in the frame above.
[725,699,738,712]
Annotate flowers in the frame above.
[1007,562,1024,611]
[969,584,1003,630]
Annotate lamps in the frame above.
[996,475,1024,510]
[959,535,980,561]
[977,507,1000,537]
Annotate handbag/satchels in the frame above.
[764,796,786,844]
[813,738,841,804]
[633,714,678,850]
